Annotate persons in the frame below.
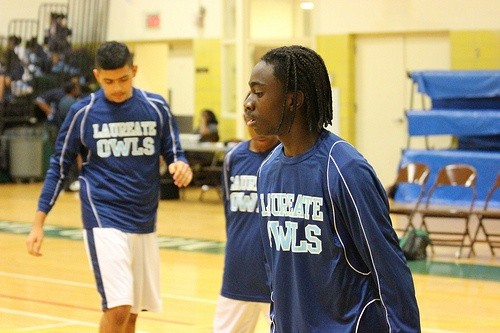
[214,93,305,333]
[26,42,193,333]
[0,13,82,120]
[244,45,422,333]
[188,110,220,158]
[55,86,83,192]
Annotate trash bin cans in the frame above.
[2,127,43,183]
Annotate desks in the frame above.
[182,142,232,201]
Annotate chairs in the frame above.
[385,162,500,260]
